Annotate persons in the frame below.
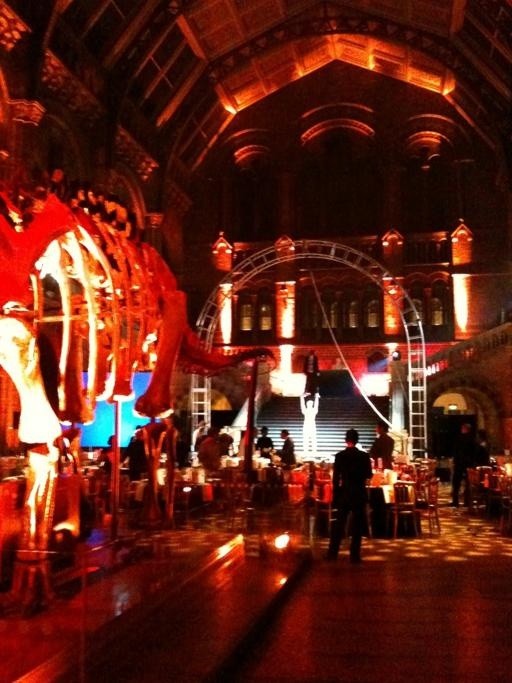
[172,413,190,466]
[94,434,114,478]
[197,425,220,471]
[369,420,394,469]
[446,421,474,506]
[123,424,146,480]
[474,429,491,465]
[254,425,274,456]
[191,419,207,450]
[276,427,295,470]
[321,427,374,561]
